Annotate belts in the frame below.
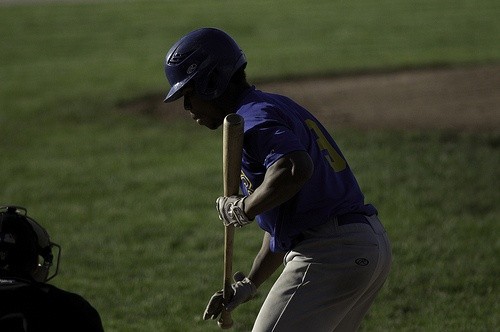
[290,214,371,248]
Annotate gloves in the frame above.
[215,194,256,229]
[203,272,258,321]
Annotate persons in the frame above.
[0,205,104,332]
[164,28,390,332]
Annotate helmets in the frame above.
[0,205,62,283]
[162,28,248,103]
